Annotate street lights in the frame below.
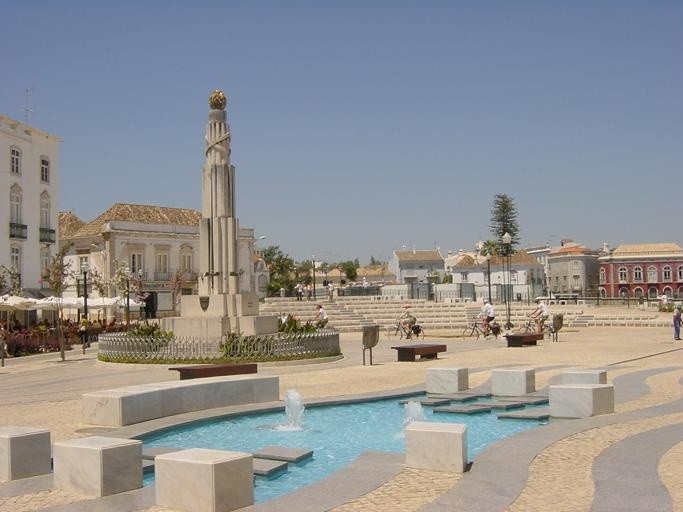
[75,260,93,347]
[246,235,266,293]
[124,266,130,328]
[312,254,315,299]
[486,252,493,305]
[137,268,144,320]
[502,232,512,328]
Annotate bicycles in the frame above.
[515,313,558,341]
[387,314,424,342]
[298,321,338,333]
[462,314,505,344]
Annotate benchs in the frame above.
[503,333,544,347]
[390,343,447,362]
[168,363,257,381]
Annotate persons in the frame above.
[671,303,683,340]
[476,298,494,336]
[293,282,313,302]
[326,280,334,302]
[398,303,416,338]
[530,298,549,334]
[656,292,669,313]
[77,314,90,350]
[311,304,328,328]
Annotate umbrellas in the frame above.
[0,294,141,332]
